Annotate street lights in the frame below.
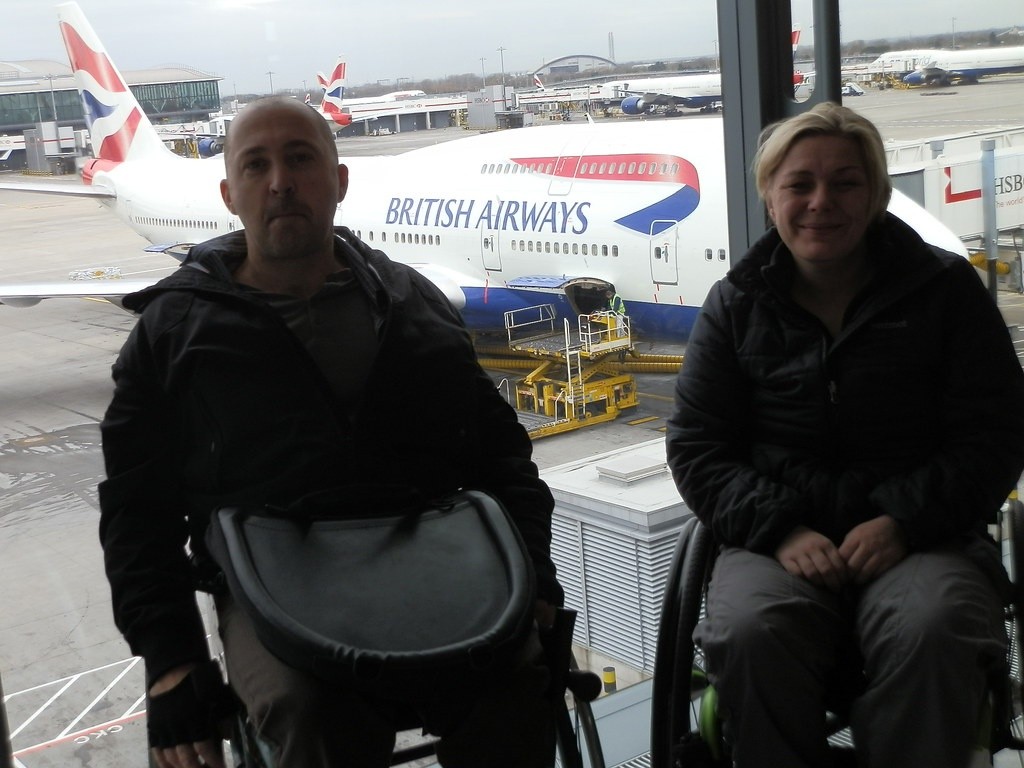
[712,40,719,68]
[480,56,486,88]
[302,78,307,94]
[266,72,276,95]
[952,17,957,50]
[497,45,507,111]
[43,73,60,121]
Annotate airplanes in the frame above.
[0,1,970,339]
[612,22,804,116]
[868,46,1024,86]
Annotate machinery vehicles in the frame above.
[471,302,684,439]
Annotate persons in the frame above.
[606,291,625,338]
[664,99,1024,768]
[99,97,564,768]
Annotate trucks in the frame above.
[842,83,863,96]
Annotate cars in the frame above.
[379,128,392,137]
